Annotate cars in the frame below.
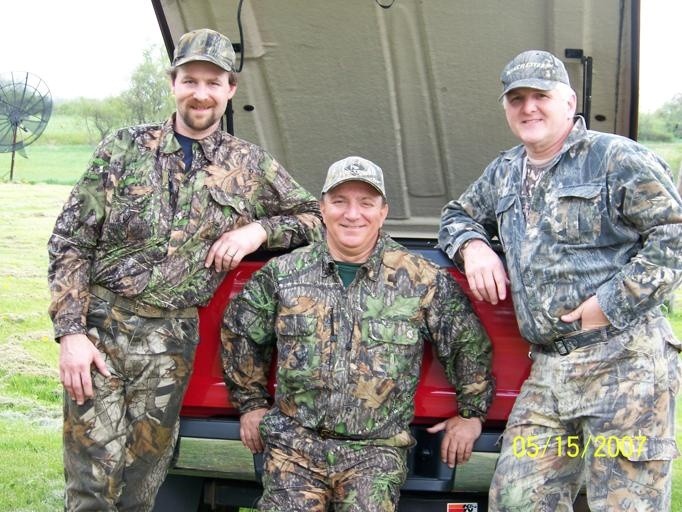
[152,0,665,511]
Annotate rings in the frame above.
[226,251,234,257]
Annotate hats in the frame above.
[172,28,236,71]
[497,50,570,100]
[322,155,387,201]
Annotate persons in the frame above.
[438,50,682,512]
[219,154,495,512]
[47,28,324,512]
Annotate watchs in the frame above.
[460,409,481,418]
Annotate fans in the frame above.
[0,70,53,182]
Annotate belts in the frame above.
[537,328,614,356]
[87,284,198,319]
[318,428,360,441]
